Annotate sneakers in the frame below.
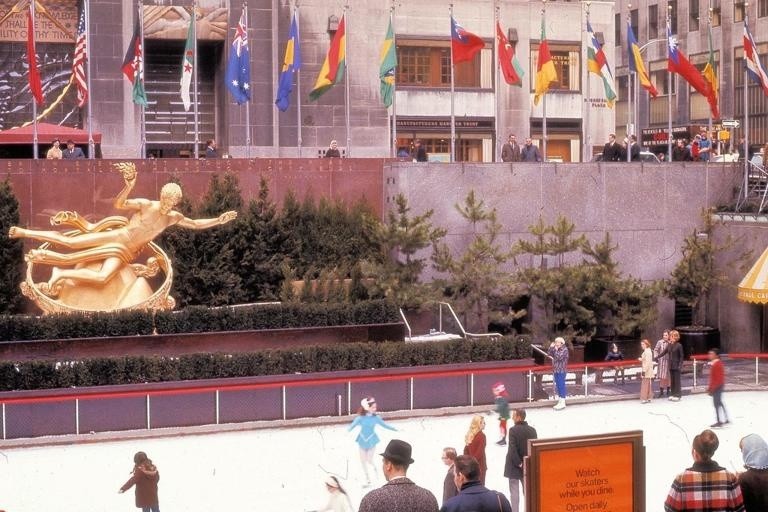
[711,420,730,427]
[668,396,680,401]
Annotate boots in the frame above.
[552,398,566,410]
[497,437,507,445]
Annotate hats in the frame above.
[378,439,416,467]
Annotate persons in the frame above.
[415,140,427,162]
[521,137,542,162]
[502,134,521,162]
[601,134,712,162]
[206,139,219,158]
[118,452,160,512]
[62,139,85,159]
[326,140,340,157]
[9,166,238,292]
[47,138,63,160]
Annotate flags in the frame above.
[225,8,251,105]
[743,21,768,96]
[72,8,88,109]
[275,10,302,113]
[28,6,44,106]
[379,17,397,108]
[120,8,149,108]
[627,22,659,99]
[703,24,719,119]
[667,26,712,97]
[587,20,616,109]
[180,12,194,112]
[534,14,558,107]
[451,15,485,65]
[309,14,345,102]
[497,21,525,88]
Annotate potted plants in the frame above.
[592,274,671,363]
[517,212,623,365]
[663,206,756,361]
